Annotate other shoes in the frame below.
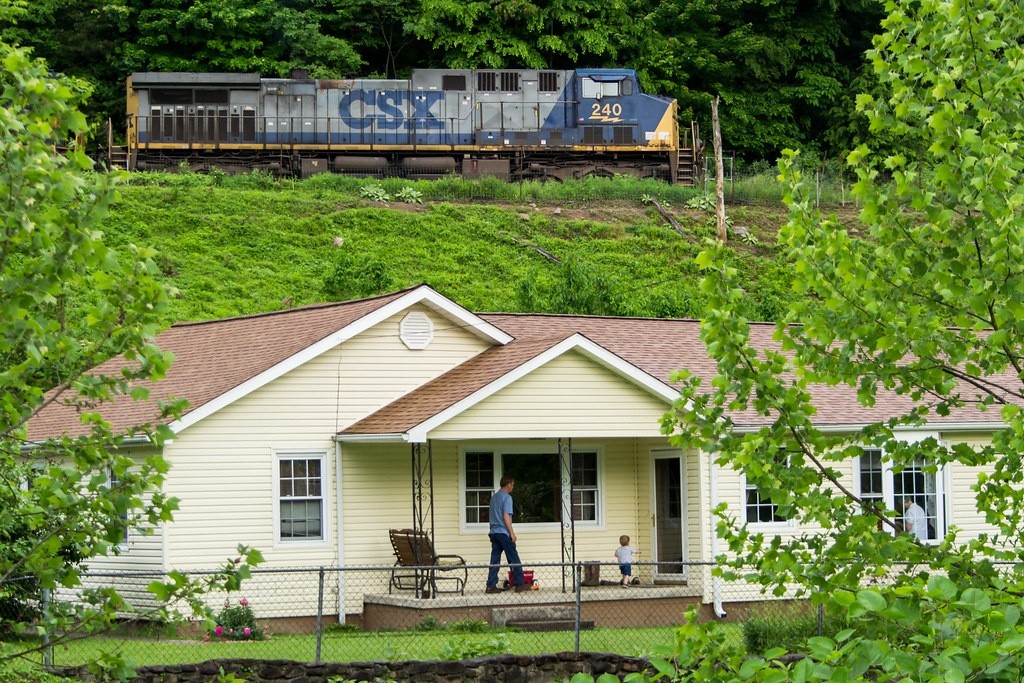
[486,586,501,595]
[514,584,530,592]
[622,583,630,588]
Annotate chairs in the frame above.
[389,528,467,593]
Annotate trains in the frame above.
[21,69,703,186]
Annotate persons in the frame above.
[614,535,636,589]
[896,493,927,541]
[484,473,531,593]
